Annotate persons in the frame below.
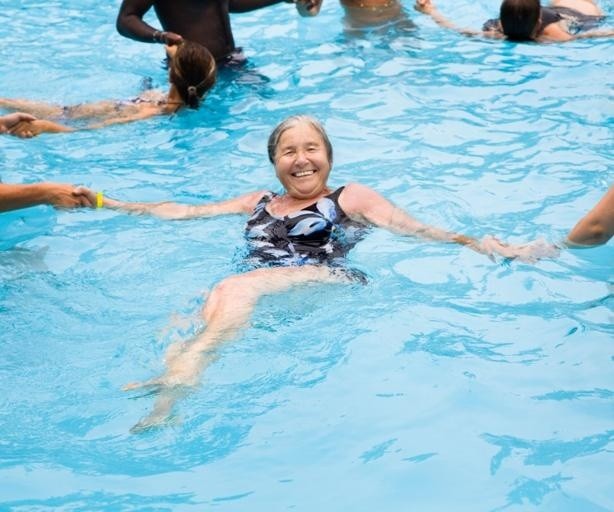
[1,111,38,134]
[296,0,434,45]
[116,0,323,101]
[71,114,562,438]
[413,0,614,45]
[479,182,614,265]
[1,40,218,140]
[1,181,94,214]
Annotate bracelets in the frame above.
[153,30,160,42]
[97,192,104,207]
